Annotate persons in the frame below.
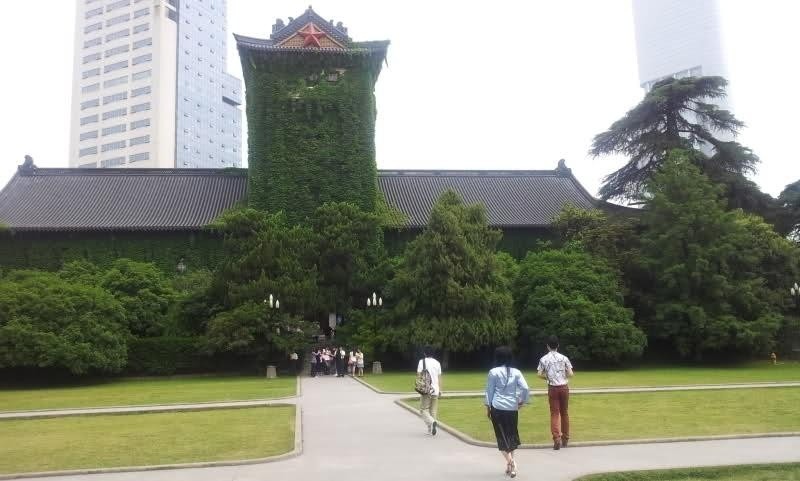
[416,346,442,437]
[535,334,574,453]
[310,346,364,378]
[483,347,529,479]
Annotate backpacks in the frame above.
[416,369,434,395]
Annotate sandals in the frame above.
[507,459,516,478]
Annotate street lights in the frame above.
[790,281,800,304]
[264,292,280,379]
[366,290,383,375]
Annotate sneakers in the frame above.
[431,421,437,435]
[554,441,560,450]
